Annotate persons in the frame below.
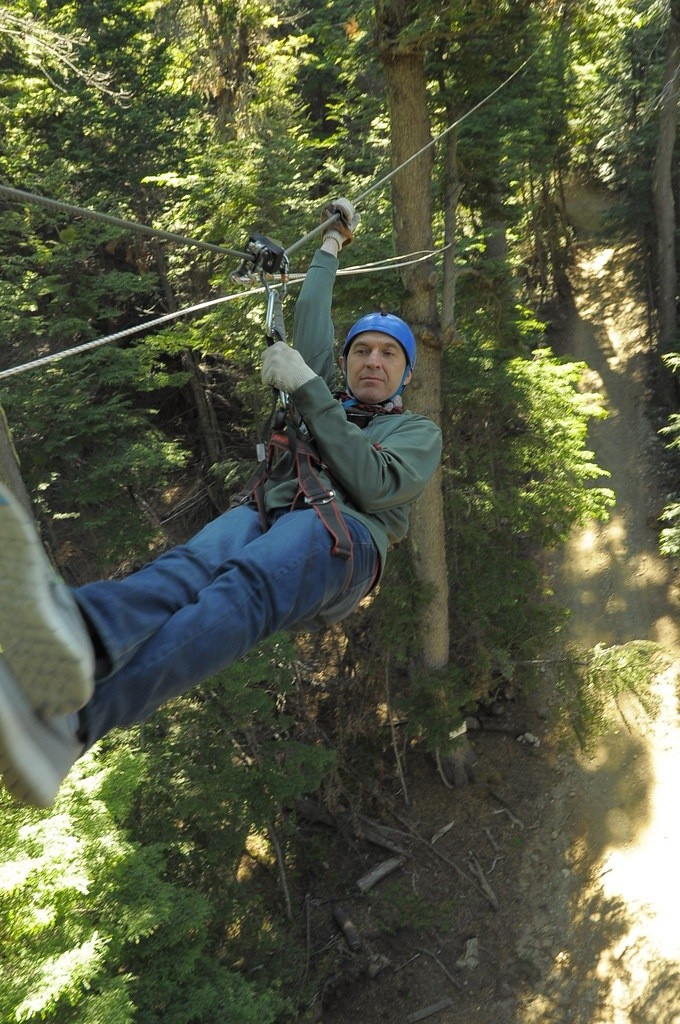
[1,197,444,810]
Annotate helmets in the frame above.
[341,311,417,372]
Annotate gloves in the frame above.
[258,342,319,393]
[319,196,361,248]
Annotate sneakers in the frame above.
[0,482,94,811]
